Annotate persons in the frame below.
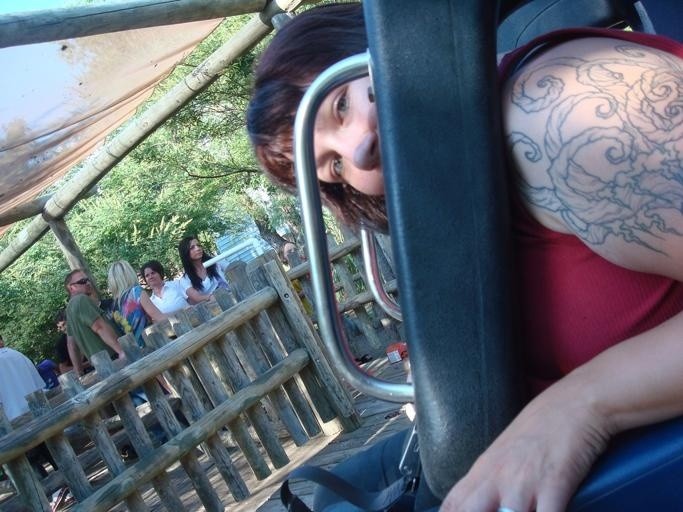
[279,241,299,273]
[177,235,230,306]
[243,1,683,512]
[108,260,173,394]
[62,268,169,449]
[90,288,125,337]
[54,310,94,375]
[0,336,77,512]
[139,258,189,315]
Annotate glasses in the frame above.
[69,277,91,288]
